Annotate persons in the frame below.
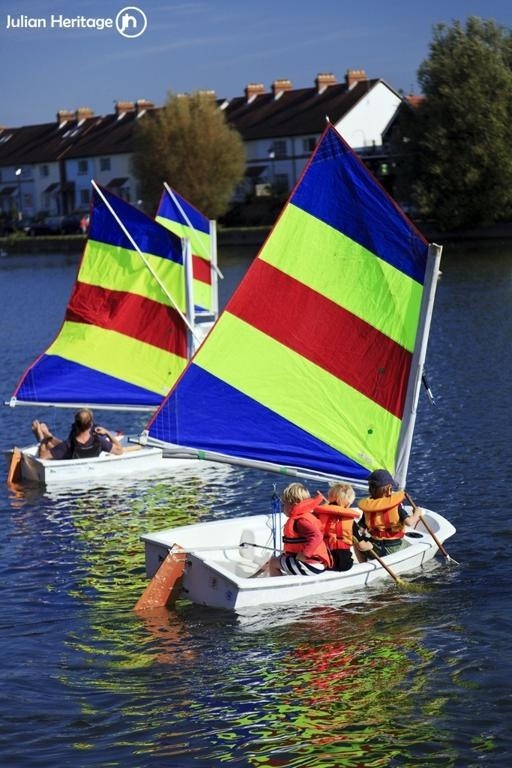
[266,479,329,576]
[352,466,423,564]
[29,408,124,460]
[311,482,375,573]
[78,214,88,233]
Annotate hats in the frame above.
[368,468,397,488]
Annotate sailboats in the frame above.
[0,111,459,612]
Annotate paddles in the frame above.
[371,549,436,592]
[404,493,461,577]
